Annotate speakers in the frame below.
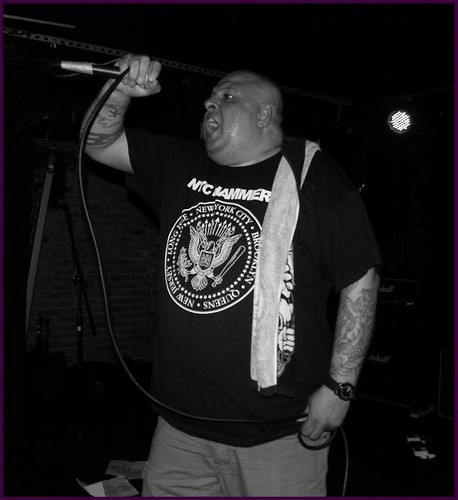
[353,298,418,412]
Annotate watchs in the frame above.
[324,376,356,401]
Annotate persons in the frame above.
[82,54,382,497]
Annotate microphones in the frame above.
[50,60,123,80]
